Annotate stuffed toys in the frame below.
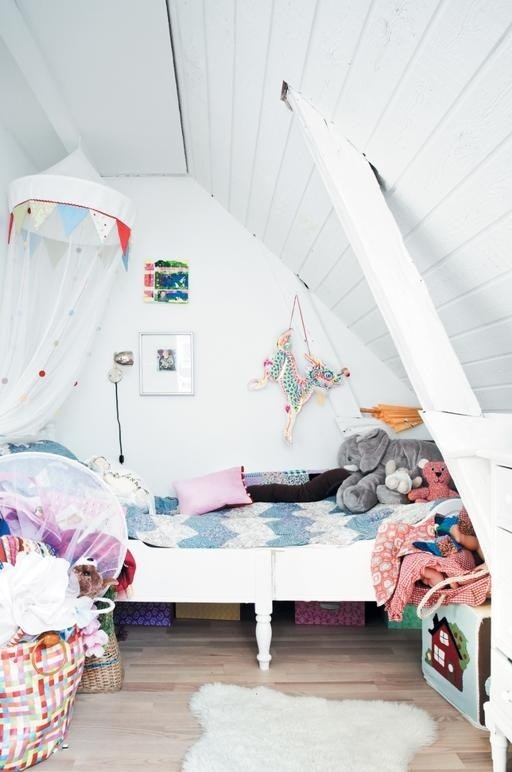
[250,328,350,447]
[70,558,119,598]
[336,428,485,590]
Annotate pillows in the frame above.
[174,467,252,514]
[7,440,80,463]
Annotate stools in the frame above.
[419,602,492,730]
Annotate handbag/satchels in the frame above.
[0,629,86,772]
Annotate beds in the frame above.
[92,457,462,669]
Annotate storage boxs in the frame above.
[175,602,241,620]
[387,605,421,629]
[113,602,169,625]
[294,601,366,626]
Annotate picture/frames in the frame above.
[139,332,193,395]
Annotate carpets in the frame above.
[181,680,438,772]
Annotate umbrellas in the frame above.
[360,402,424,433]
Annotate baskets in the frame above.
[77,632,122,693]
